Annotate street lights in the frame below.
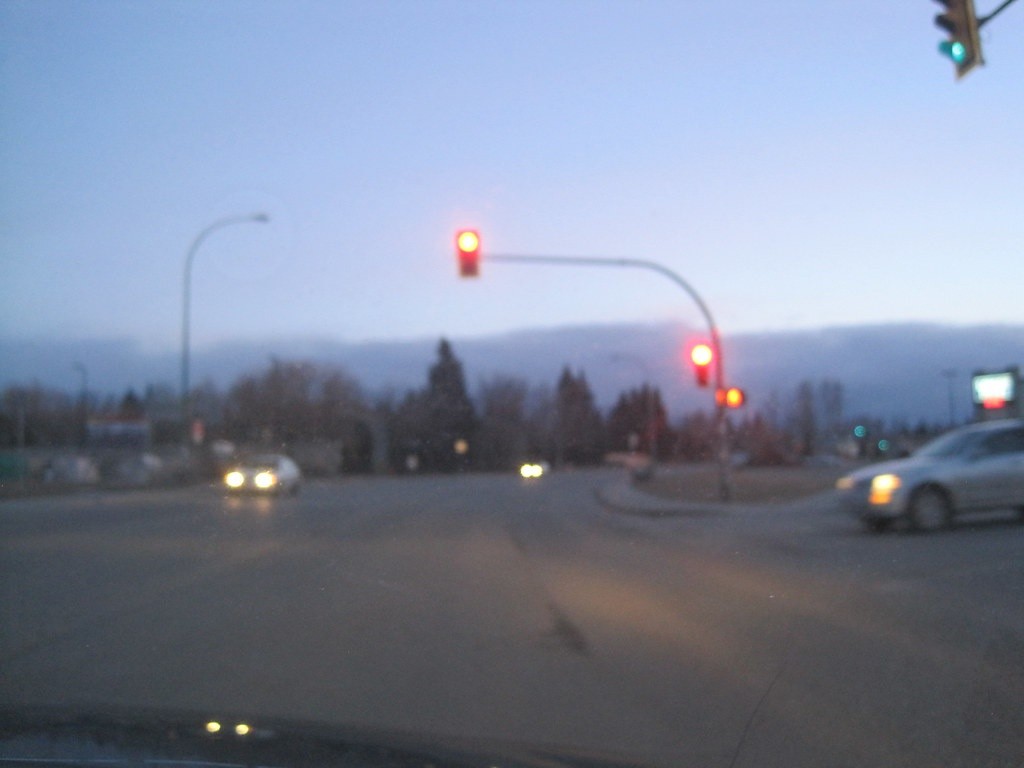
[182,214,268,412]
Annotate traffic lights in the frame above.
[934,0,982,77]
[456,229,478,276]
[728,389,743,406]
[695,345,710,385]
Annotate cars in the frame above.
[232,453,303,495]
[835,418,1024,534]
